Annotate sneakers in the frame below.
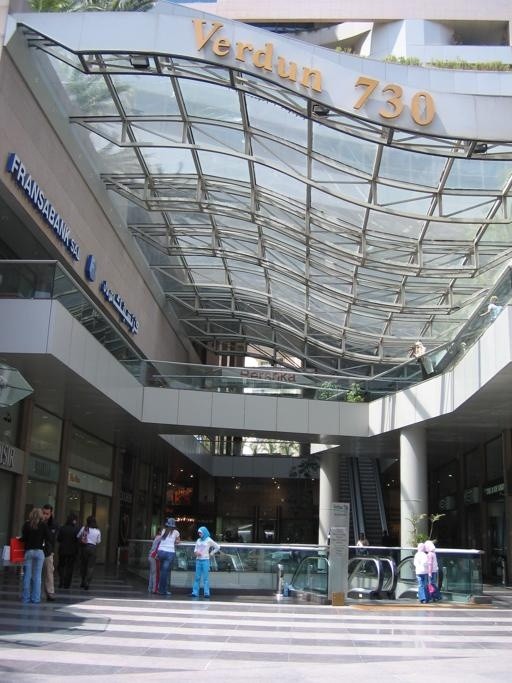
[419,596,442,604]
[188,593,210,598]
[23,594,56,604]
[147,589,172,595]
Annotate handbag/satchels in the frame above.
[9,537,25,563]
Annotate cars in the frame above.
[238,524,274,542]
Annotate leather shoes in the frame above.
[59,583,70,589]
[80,581,89,589]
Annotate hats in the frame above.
[165,518,177,528]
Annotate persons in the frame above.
[148,518,181,595]
[187,526,220,597]
[381,530,391,547]
[356,533,370,555]
[414,540,444,603]
[16,504,101,603]
[409,342,434,380]
[480,296,503,322]
